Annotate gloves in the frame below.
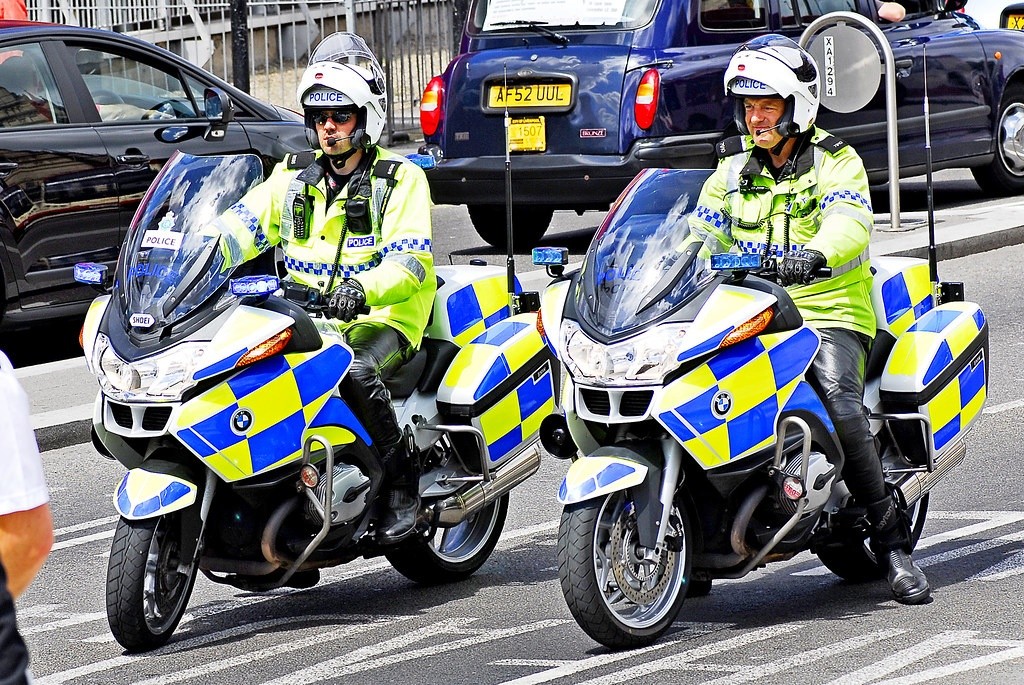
[779,249,826,286]
[326,278,366,323]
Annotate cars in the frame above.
[405,0,1024,251]
[0,19,320,338]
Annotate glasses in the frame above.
[308,109,359,125]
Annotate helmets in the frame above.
[724,34,820,155]
[298,32,387,168]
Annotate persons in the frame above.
[199,31,438,544]
[94,103,178,124]
[671,32,931,605]
[801,0,906,23]
[0,56,39,96]
[0,349,54,685]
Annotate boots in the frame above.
[865,492,930,605]
[378,426,421,541]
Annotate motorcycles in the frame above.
[74,63,565,653]
[532,43,991,651]
